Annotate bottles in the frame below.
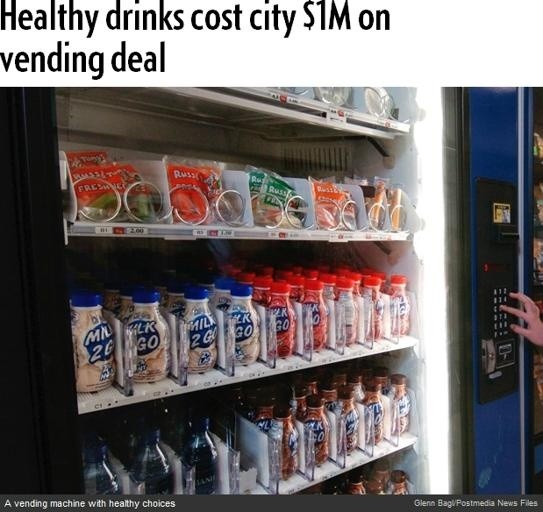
[216,356,411,497]
[78,398,220,496]
[64,256,412,394]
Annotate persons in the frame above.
[498,289,543,347]
